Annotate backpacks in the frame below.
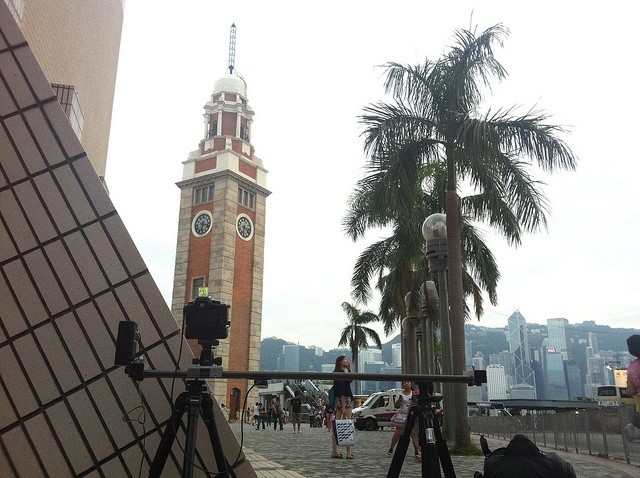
[328,384,336,405]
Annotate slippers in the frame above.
[332,453,344,459]
[346,454,356,459]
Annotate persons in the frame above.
[265,404,271,427]
[221,403,230,420]
[387,380,420,460]
[255,402,266,431]
[286,408,289,423]
[330,355,355,459]
[295,379,305,386]
[309,387,327,427]
[626,335,639,429]
[282,407,286,424]
[271,397,284,430]
[247,406,250,423]
[267,379,283,384]
[250,409,253,423]
[326,386,336,431]
[290,391,302,434]
[236,408,240,421]
[254,401,272,418]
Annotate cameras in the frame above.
[181,294,232,343]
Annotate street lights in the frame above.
[420,280,440,376]
[421,211,453,445]
[404,291,419,374]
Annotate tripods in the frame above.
[147,388,232,478]
[387,401,457,477]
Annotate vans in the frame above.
[351,389,443,432]
[597,384,635,405]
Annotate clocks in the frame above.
[191,210,213,238]
[236,213,254,241]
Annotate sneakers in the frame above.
[414,451,421,462]
[387,448,394,457]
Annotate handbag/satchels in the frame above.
[389,411,409,427]
[332,414,356,447]
